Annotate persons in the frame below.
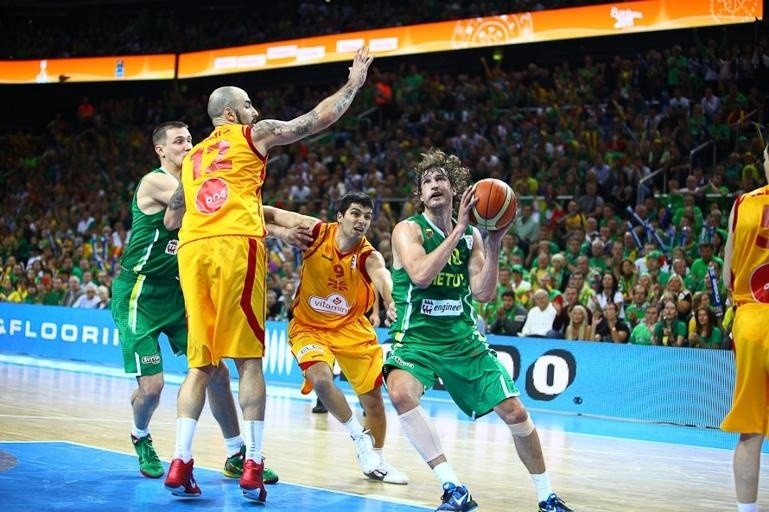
[0,83,110,310]
[266,268,301,320]
[110,120,315,486]
[475,196,576,339]
[443,150,577,195]
[262,121,423,268]
[262,190,408,487]
[728,38,768,239]
[719,141,769,511]
[110,80,161,260]
[376,0,577,149]
[381,149,576,512]
[162,0,376,146]
[162,42,376,504]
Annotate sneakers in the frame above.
[433,485,478,512]
[223,446,279,485]
[164,458,201,497]
[350,428,378,472]
[537,493,574,512]
[364,463,409,485]
[130,431,165,479]
[239,458,268,502]
[312,397,328,414]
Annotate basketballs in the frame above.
[469,177,518,232]
